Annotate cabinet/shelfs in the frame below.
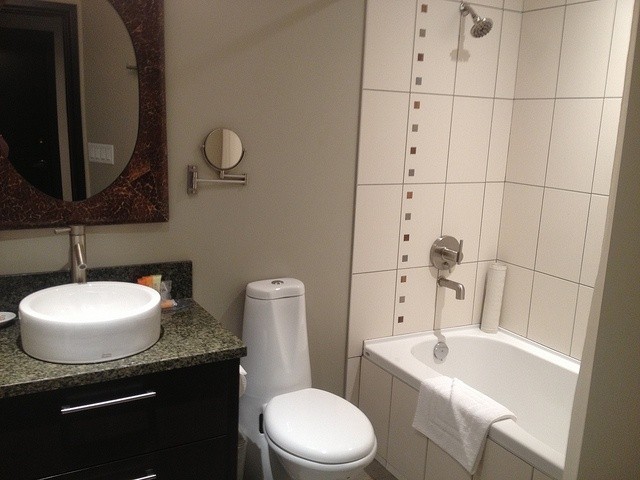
[1,362,238,480]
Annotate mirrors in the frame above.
[1,0,171,231]
[203,129,245,171]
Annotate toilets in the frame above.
[237,277,377,480]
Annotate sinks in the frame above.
[18,280,161,365]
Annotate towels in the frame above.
[410,373,518,475]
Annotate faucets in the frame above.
[439,278,465,300]
[53,224,86,283]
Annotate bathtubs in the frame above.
[358,324,581,480]
